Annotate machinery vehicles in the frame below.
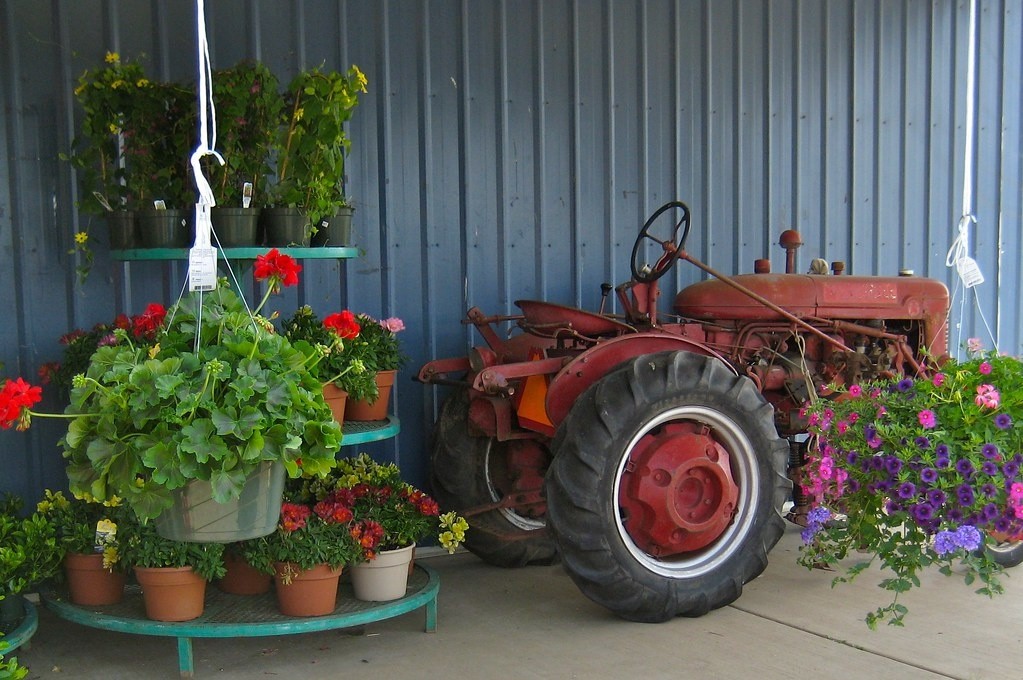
[410,201,950,625]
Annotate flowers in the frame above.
[781,338,1023,630]
[0,49,471,587]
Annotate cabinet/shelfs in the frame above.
[39,246,441,678]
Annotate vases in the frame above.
[351,539,417,603]
[210,209,258,247]
[311,205,354,247]
[273,561,344,616]
[214,548,271,595]
[133,566,205,622]
[106,211,140,248]
[139,211,193,248]
[64,553,123,606]
[303,383,349,427]
[341,370,398,421]
[153,451,291,544]
[258,209,308,247]
